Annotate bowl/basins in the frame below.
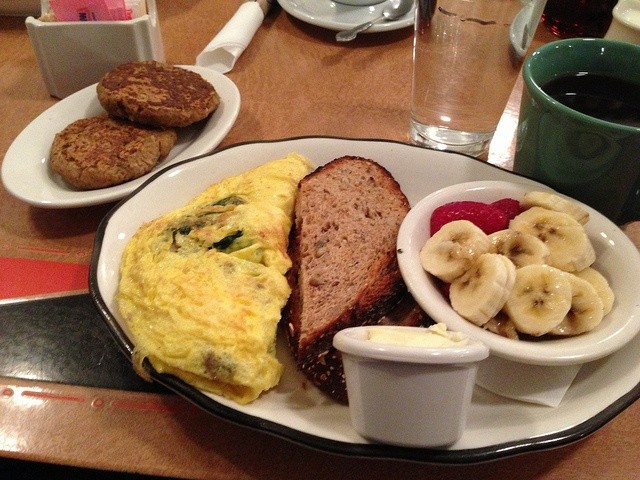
[331,323,492,449]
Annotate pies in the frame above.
[97,60,219,127]
[114,151,313,405]
[50,113,178,189]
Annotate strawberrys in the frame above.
[430,202,506,240]
[489,199,521,220]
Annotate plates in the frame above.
[613,0,640,34]
[1,64,241,212]
[394,180,639,364]
[89,133,640,459]
[509,1,544,59]
[276,0,421,34]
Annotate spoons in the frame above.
[336,0,414,44]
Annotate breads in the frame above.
[287,156,428,405]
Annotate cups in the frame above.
[543,0,619,39]
[410,1,546,158]
[514,38,640,229]
